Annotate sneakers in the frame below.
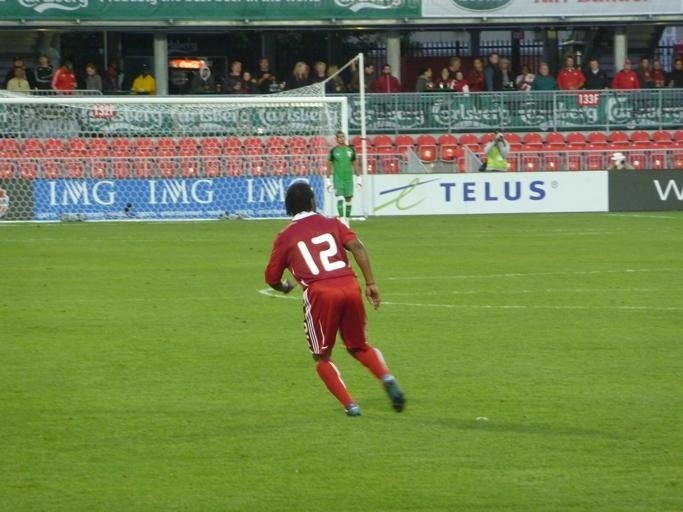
[384,376,404,412]
[343,404,359,415]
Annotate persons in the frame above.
[483,130,510,172]
[263,183,405,416]
[191,57,283,94]
[289,58,401,93]
[5,54,127,95]
[516,56,608,91]
[606,152,635,170]
[133,66,156,95]
[326,130,362,218]
[612,58,683,89]
[416,54,514,92]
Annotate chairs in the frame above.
[3,135,333,177]
[350,129,683,175]
